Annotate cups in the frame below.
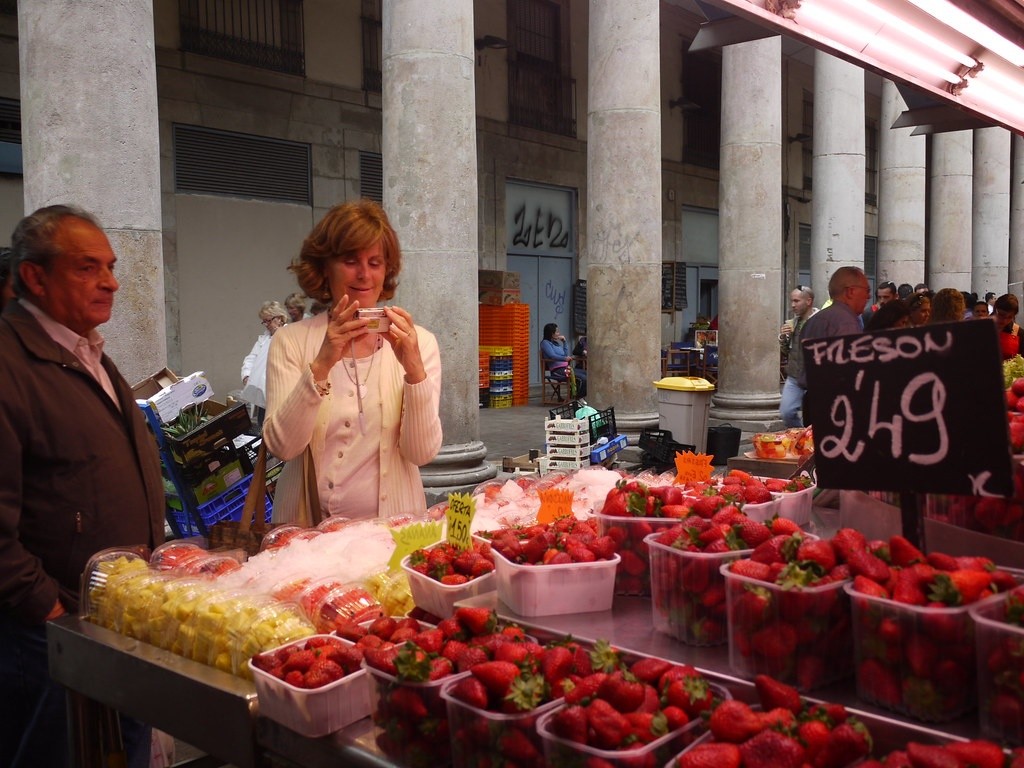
[786,320,795,332]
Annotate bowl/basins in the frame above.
[245,429,1024,768]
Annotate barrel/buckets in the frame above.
[707,423,741,465]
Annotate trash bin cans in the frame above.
[651,376,716,454]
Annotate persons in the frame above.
[0,205,166,768]
[240,293,332,427]
[262,202,443,523]
[540,323,587,405]
[778,266,1024,429]
[0,247,21,312]
[573,327,588,357]
[696,312,718,330]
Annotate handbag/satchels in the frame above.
[206,437,321,559]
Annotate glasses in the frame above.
[796,284,811,295]
[842,286,871,293]
[261,317,275,326]
[994,308,1015,320]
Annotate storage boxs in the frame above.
[79,270,1024,768]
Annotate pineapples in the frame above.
[366,571,414,620]
[88,555,317,684]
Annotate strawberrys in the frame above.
[408,515,614,587]
[252,606,1024,768]
[649,494,1024,749]
[491,470,807,597]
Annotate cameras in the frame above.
[352,308,391,333]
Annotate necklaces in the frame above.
[341,332,379,386]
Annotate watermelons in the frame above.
[157,516,384,636]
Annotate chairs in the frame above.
[538,349,586,405]
[662,342,720,387]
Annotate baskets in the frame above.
[638,427,696,472]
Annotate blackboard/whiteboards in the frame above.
[801,318,1015,494]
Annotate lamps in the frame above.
[475,34,509,49]
[669,97,700,109]
[790,133,812,143]
